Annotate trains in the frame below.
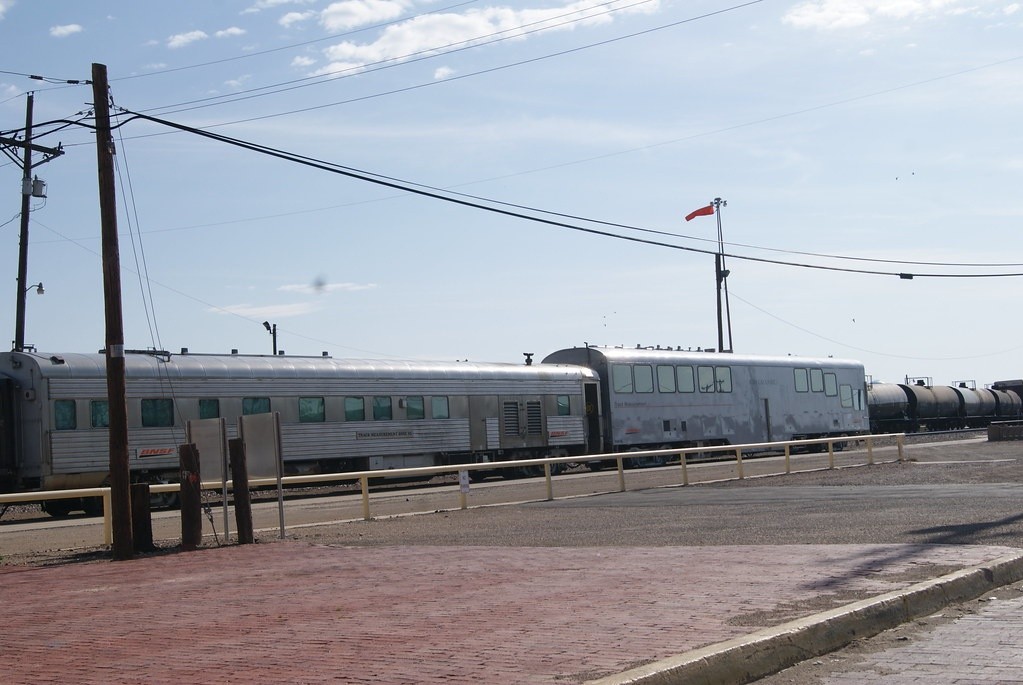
[1,340,873,520]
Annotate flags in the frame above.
[685,206,714,221]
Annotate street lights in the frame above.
[14,282,46,352]
[263,321,279,355]
